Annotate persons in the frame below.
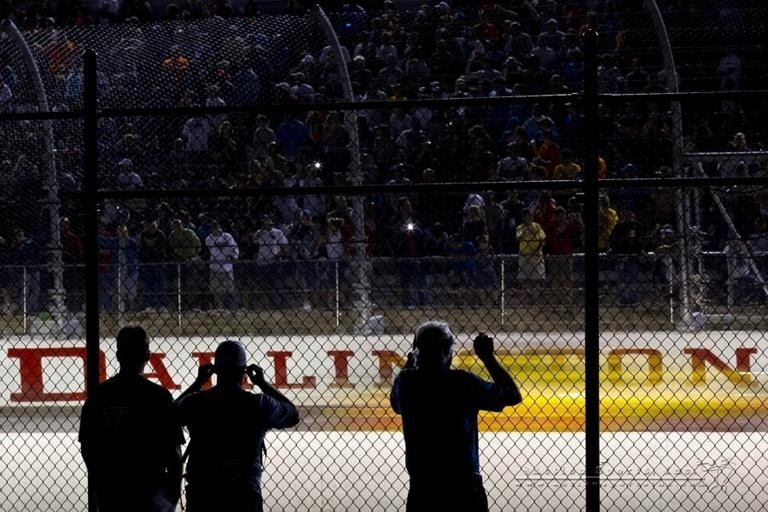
[175,342,299,511]
[389,319,521,511]
[78,324,186,512]
[0,1,767,316]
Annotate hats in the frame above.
[213,340,247,367]
[118,158,133,166]
